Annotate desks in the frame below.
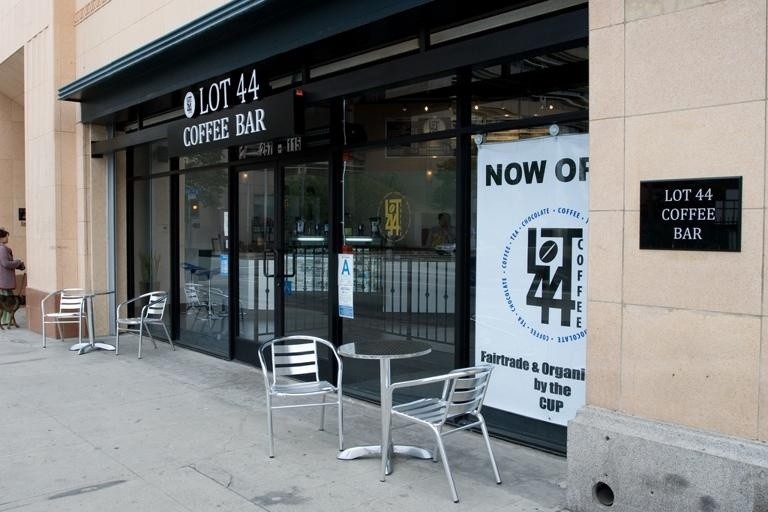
[337,339,433,483]
[63,289,116,354]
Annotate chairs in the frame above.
[41,289,87,348]
[116,290,176,359]
[184,283,248,340]
[257,335,343,456]
[380,364,502,503]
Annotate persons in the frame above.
[425,212,456,248]
[0,229,26,325]
